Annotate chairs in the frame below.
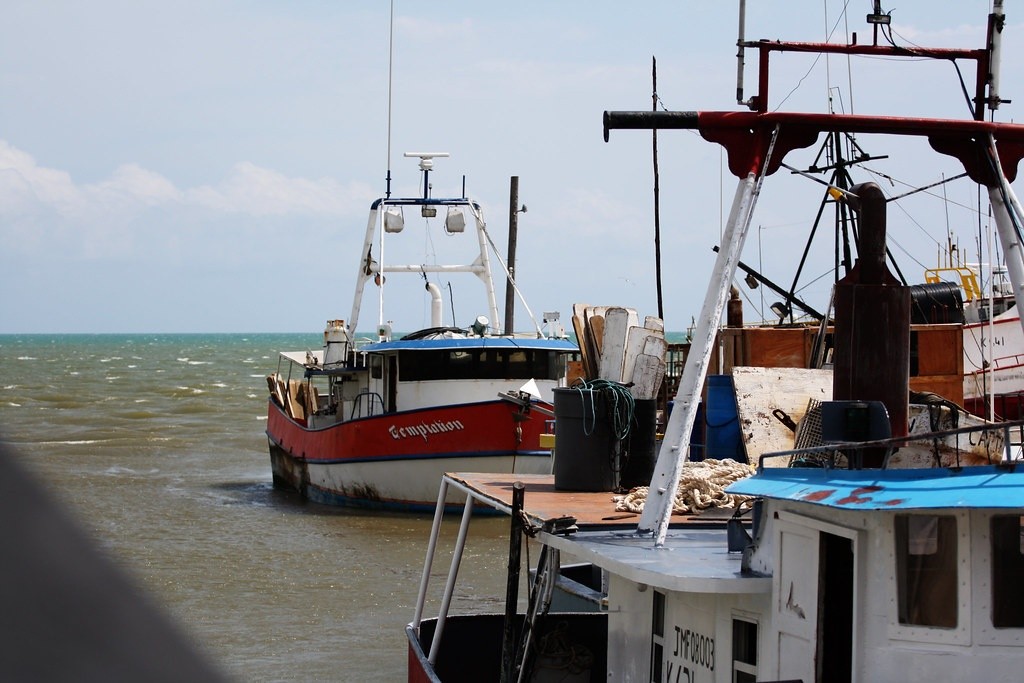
[794,398,892,468]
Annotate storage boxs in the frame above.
[888,404,1007,469]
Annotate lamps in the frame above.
[770,302,791,318]
[444,205,465,233]
[377,325,392,343]
[422,205,436,217]
[468,316,490,338]
[745,274,759,288]
[727,497,764,551]
[384,203,404,234]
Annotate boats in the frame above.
[406,0,1024,683]
[262,0,582,510]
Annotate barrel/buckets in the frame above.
[551,387,620,490]
[620,399,657,490]
[705,374,746,463]
